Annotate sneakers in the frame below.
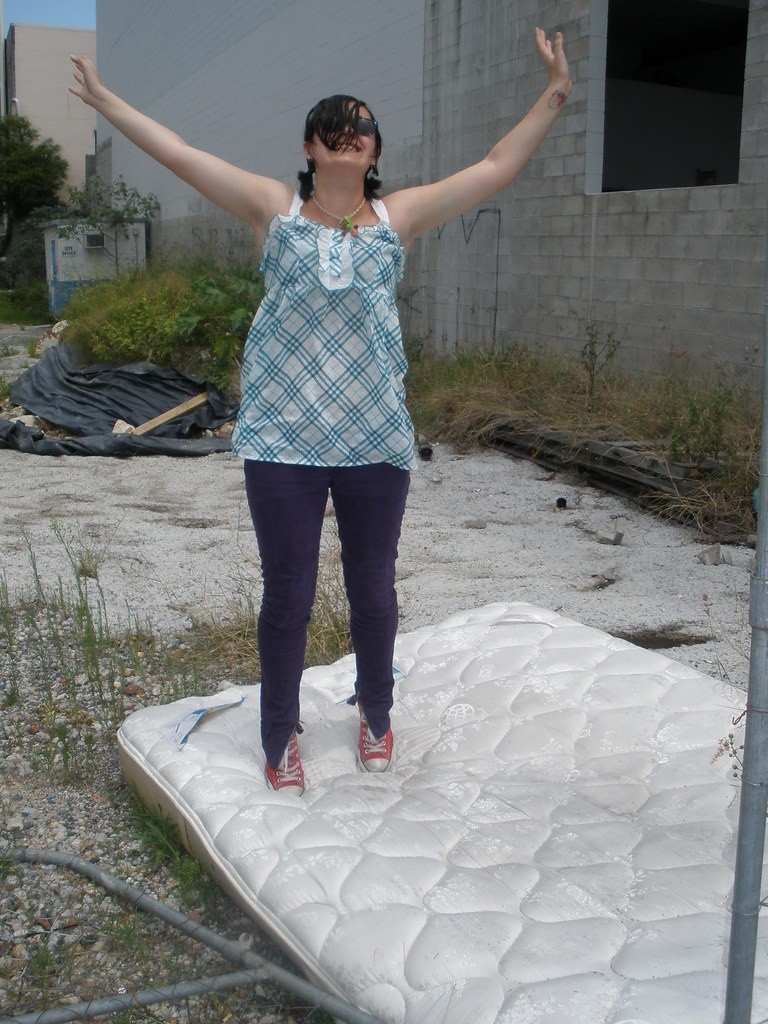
[356,698,395,773]
[265,727,304,797]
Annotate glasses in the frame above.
[320,113,379,141]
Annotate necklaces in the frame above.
[313,194,366,237]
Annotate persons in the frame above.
[69,28,574,797]
